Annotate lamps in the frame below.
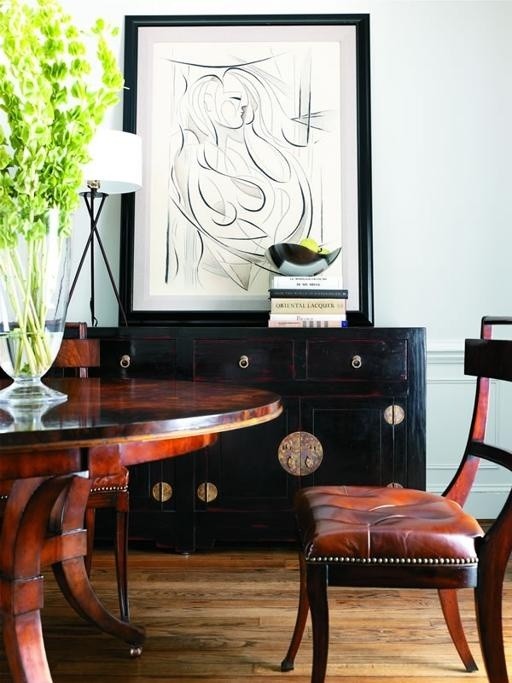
[67,126,140,329]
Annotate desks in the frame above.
[0,374,282,683]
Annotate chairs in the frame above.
[36,323,131,625]
[282,315,512,683]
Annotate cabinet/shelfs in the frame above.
[0,324,183,551]
[192,326,429,553]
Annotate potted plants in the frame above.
[1,0,124,402]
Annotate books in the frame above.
[268,275,349,328]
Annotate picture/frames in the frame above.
[124,14,375,328]
